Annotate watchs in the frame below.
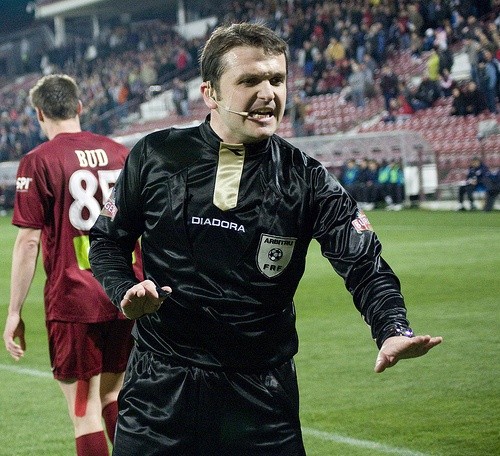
[381,323,415,345]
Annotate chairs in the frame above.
[0,1,500,211]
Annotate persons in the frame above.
[3,74,144,456]
[88,22,443,456]
[0,0,500,217]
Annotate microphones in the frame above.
[215,102,249,117]
